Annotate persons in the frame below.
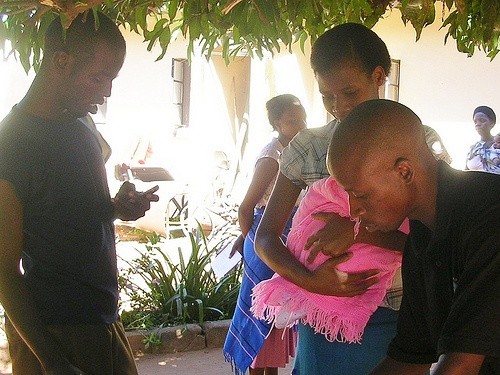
[464,106,500,174]
[223,95,309,375]
[328,99,500,375]
[0,7,159,375]
[254,22,451,375]
